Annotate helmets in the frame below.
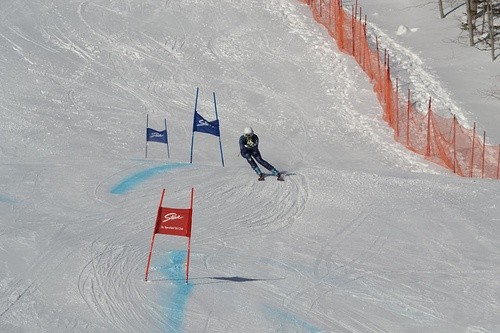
[243,127,254,138]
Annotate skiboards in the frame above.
[254,165,286,182]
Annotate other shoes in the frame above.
[258,174,265,181]
[276,174,284,180]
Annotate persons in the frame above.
[238,125,280,177]
